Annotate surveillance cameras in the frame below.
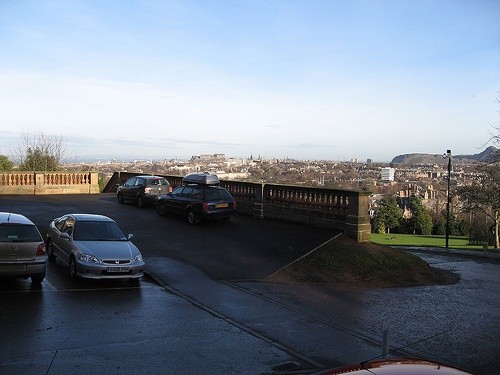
[443,155,446,159]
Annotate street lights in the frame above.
[442,150,452,249]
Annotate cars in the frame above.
[46,214,145,283]
[117,176,174,208]
[157,173,237,225]
[0,212,49,285]
